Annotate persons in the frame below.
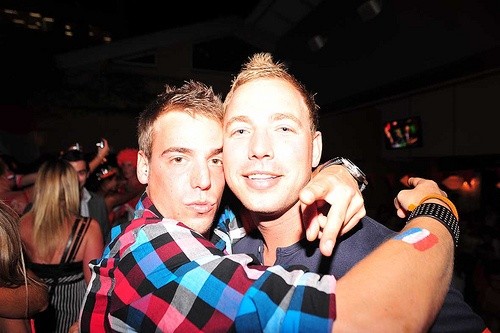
[68,51,494,333]
[442,169,478,209]
[1,139,146,333]
[78,79,460,332]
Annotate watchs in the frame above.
[318,157,368,192]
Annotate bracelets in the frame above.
[407,203,460,247]
[408,193,459,221]
